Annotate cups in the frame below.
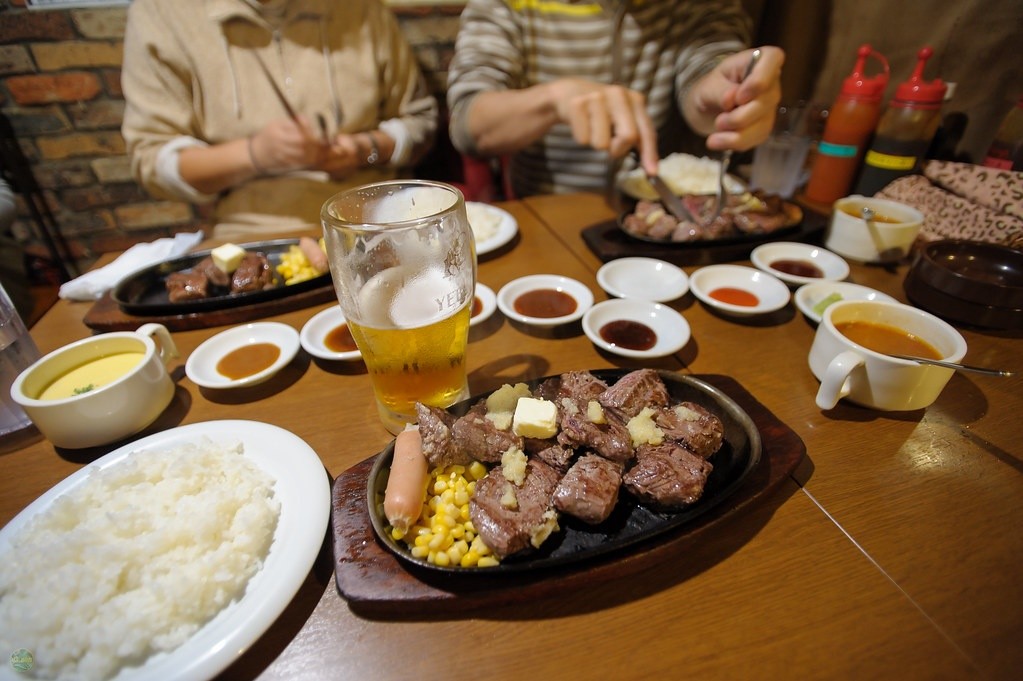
[11,324,179,450]
[320,177,480,435]
[749,101,808,199]
[808,297,967,413]
[824,195,925,263]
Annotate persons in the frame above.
[119,0,438,240]
[448,0,786,200]
[775,0,1023,165]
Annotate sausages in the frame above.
[383,423,430,534]
[299,236,329,273]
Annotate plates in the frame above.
[689,264,792,317]
[596,256,690,303]
[466,196,520,258]
[110,237,344,315]
[580,297,691,358]
[464,282,497,325]
[365,367,766,577]
[299,305,365,363]
[622,192,805,243]
[750,241,850,285]
[794,283,902,325]
[0,419,333,680]
[496,273,595,328]
[184,322,300,392]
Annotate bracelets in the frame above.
[355,131,380,169]
[247,134,274,176]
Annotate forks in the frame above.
[704,49,764,231]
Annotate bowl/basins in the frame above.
[904,238,1023,330]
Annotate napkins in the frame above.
[58,228,203,302]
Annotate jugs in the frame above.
[0,283,44,436]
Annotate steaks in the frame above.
[417,369,725,562]
[629,192,801,240]
[165,251,275,302]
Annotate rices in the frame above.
[1,435,284,681]
[467,209,500,242]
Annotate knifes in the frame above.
[618,141,699,226]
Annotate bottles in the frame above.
[854,47,952,199]
[807,46,891,205]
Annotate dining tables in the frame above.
[0,189,1023,681]
